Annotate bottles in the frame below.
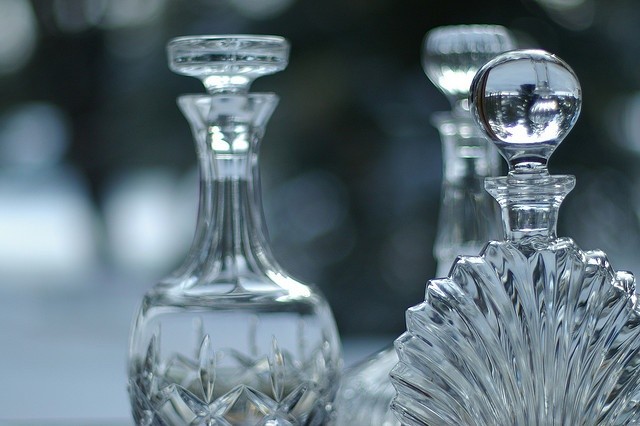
[127,35,342,424]
[393,47,639,425]
[337,23,519,424]
